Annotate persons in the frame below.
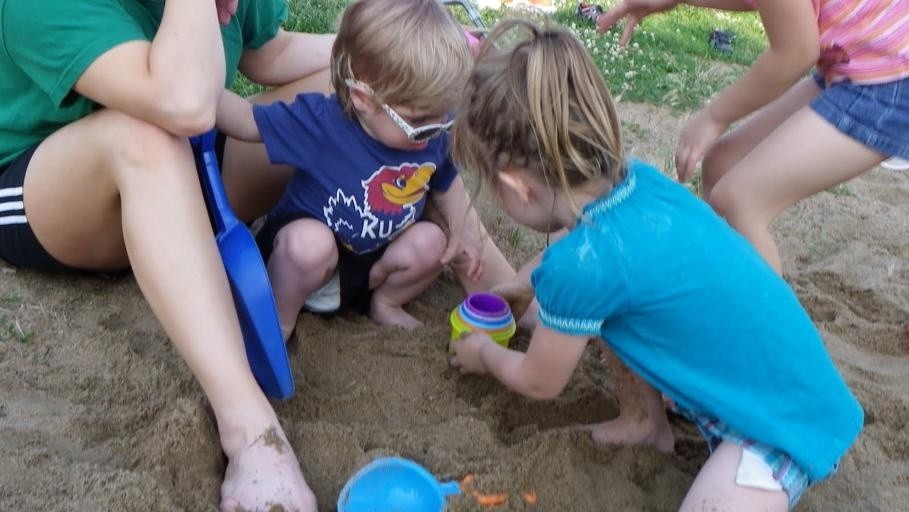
[1,0,540,512]
[215,0,487,345]
[449,17,864,512]
[595,1,909,278]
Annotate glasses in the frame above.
[341,76,456,143]
